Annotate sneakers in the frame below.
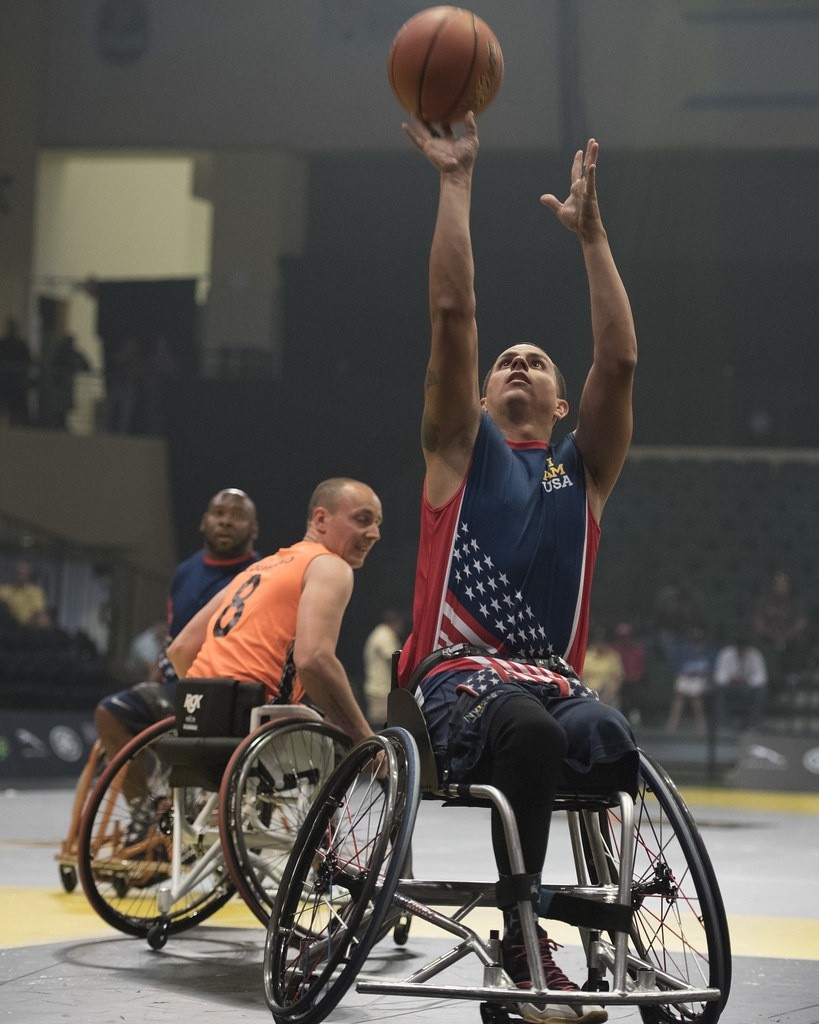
[499,924,608,1024]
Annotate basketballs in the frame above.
[386,3,507,123]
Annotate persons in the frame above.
[92,486,262,859]
[165,477,390,892]
[396,105,642,1007]
[2,558,50,630]
[358,570,808,738]
[121,615,166,681]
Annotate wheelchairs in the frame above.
[262,648,734,1023]
[55,672,416,964]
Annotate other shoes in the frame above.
[121,796,171,865]
